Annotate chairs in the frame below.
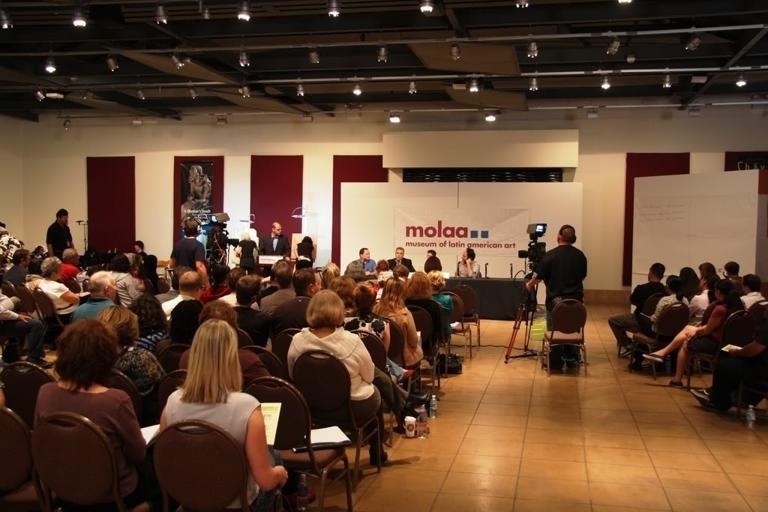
[614,292,767,420]
[537,298,591,378]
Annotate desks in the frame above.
[446,276,534,323]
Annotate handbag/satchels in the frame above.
[437,354,462,374]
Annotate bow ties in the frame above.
[272,236,279,240]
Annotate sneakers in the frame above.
[370,453,387,465]
[620,348,632,358]
[403,369,416,381]
[668,381,683,388]
[703,402,727,415]
[25,358,53,368]
[642,353,664,363]
[690,388,710,406]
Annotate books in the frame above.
[288,426,353,453]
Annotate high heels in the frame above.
[288,491,316,509]
[405,390,431,418]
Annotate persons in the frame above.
[605,248,768,413]
[0,210,479,469]
[525,225,587,378]
[157,317,317,510]
[29,318,154,511]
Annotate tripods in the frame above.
[503,269,545,364]
